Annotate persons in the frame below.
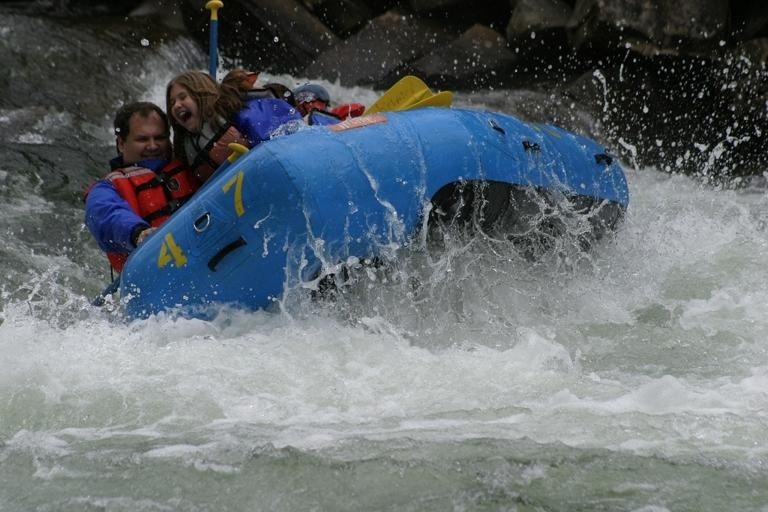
[166,72,303,179]
[85,102,204,274]
[221,69,365,124]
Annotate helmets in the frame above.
[262,83,331,108]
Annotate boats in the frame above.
[118,108,628,319]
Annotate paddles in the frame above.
[363,76,434,114]
[401,90,454,110]
